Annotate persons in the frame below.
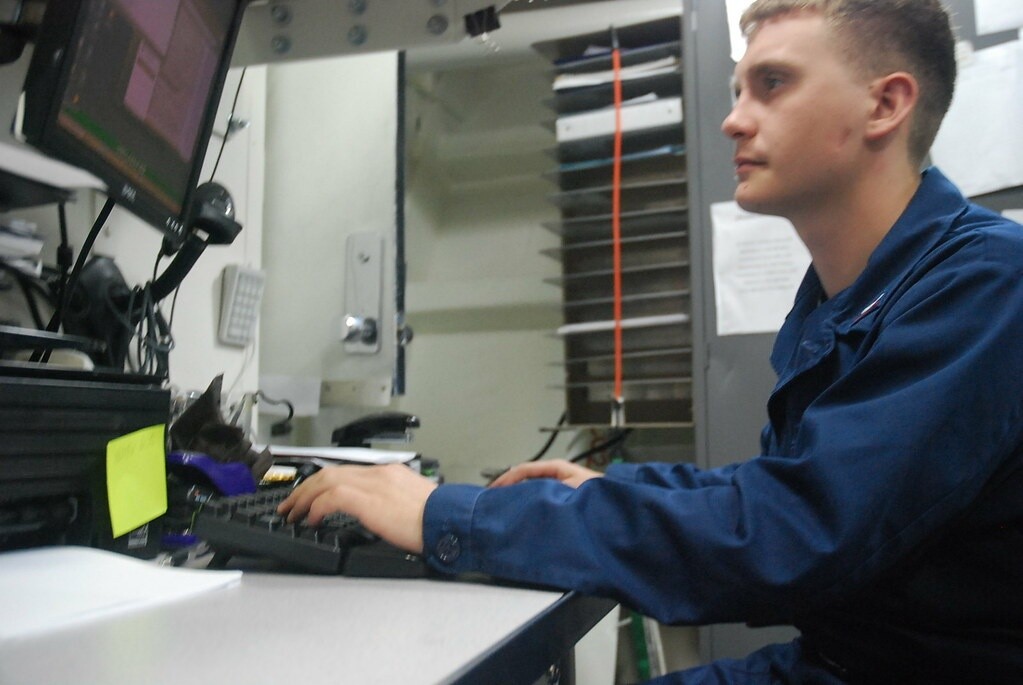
[277,1,1023,685]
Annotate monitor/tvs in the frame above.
[20,0,246,242]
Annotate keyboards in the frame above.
[196,479,435,579]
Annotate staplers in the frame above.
[332,412,441,475]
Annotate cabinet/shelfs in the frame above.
[528,14,696,432]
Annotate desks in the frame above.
[0,541,622,685]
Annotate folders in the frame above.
[555,96,683,142]
